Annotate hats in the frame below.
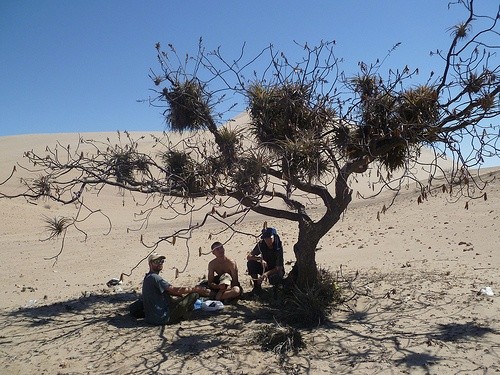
[148,253,166,262]
[261,228,275,239]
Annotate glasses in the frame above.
[153,259,164,264]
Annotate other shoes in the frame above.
[251,285,261,294]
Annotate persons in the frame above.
[142,253,207,325]
[247,227,286,294]
[195,242,243,300]
